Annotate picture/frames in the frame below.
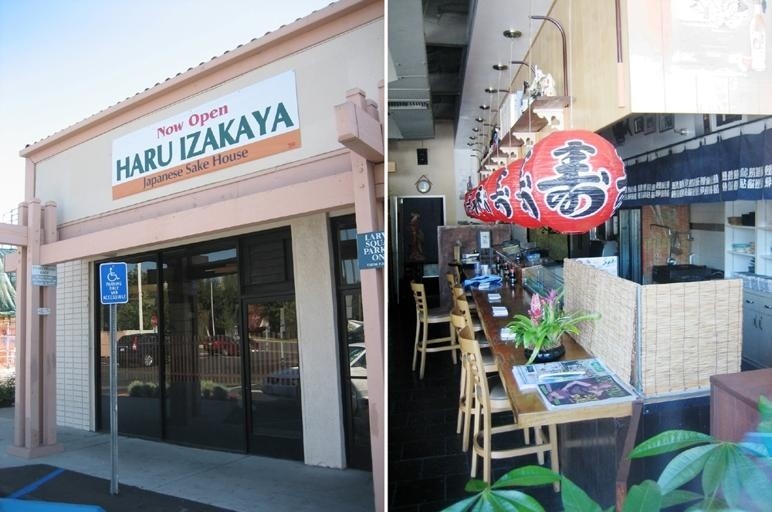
[633,116,644,134]
[716,115,742,128]
[644,116,655,136]
[659,114,675,133]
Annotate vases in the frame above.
[525,346,565,362]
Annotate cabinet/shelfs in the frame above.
[724,199,772,369]
[710,367,772,512]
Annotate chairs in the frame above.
[445,271,560,492]
[411,279,460,379]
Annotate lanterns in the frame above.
[463,130,628,234]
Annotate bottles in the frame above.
[509,267,517,289]
[503,262,511,280]
[495,256,502,273]
[515,253,521,265]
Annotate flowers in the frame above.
[505,286,595,368]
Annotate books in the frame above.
[512,357,638,411]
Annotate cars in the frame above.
[263,341,370,414]
[115,333,170,366]
[204,334,241,356]
